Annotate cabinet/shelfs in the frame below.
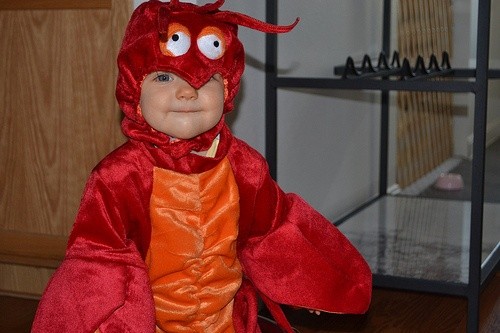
[263,0,500,333]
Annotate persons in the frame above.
[30,1,375,333]
